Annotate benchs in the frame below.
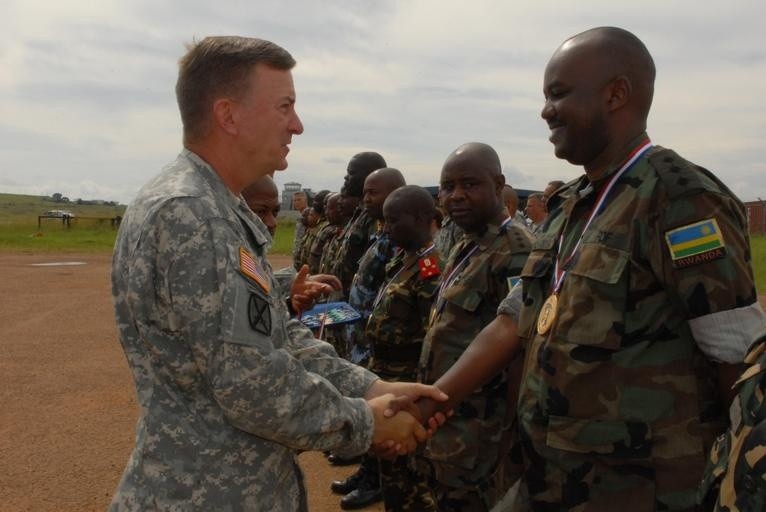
[36,214,121,228]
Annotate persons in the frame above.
[240,27,764,511]
[106,35,455,511]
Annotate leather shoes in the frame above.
[332,473,382,509]
